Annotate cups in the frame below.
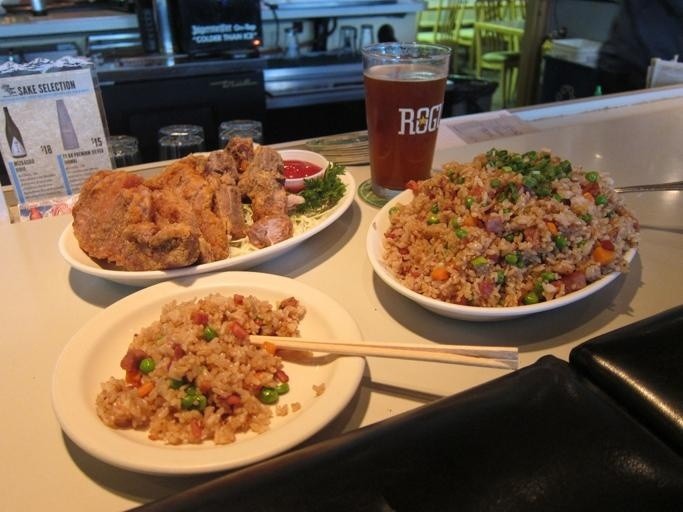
[108,120,264,168]
[339,24,356,52]
[360,41,452,200]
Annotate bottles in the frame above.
[57,99,79,151]
[361,25,373,50]
[3,107,27,158]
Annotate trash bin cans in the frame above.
[538,38,612,103]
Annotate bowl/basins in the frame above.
[274,148,334,194]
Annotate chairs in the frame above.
[417,0,551,110]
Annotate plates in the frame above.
[58,146,358,289]
[51,269,368,475]
[365,169,640,322]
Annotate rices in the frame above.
[382,150,641,307]
[96,293,326,444]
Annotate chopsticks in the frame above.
[248,334,520,372]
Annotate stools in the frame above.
[569,304,683,455]
[119,354,683,512]
